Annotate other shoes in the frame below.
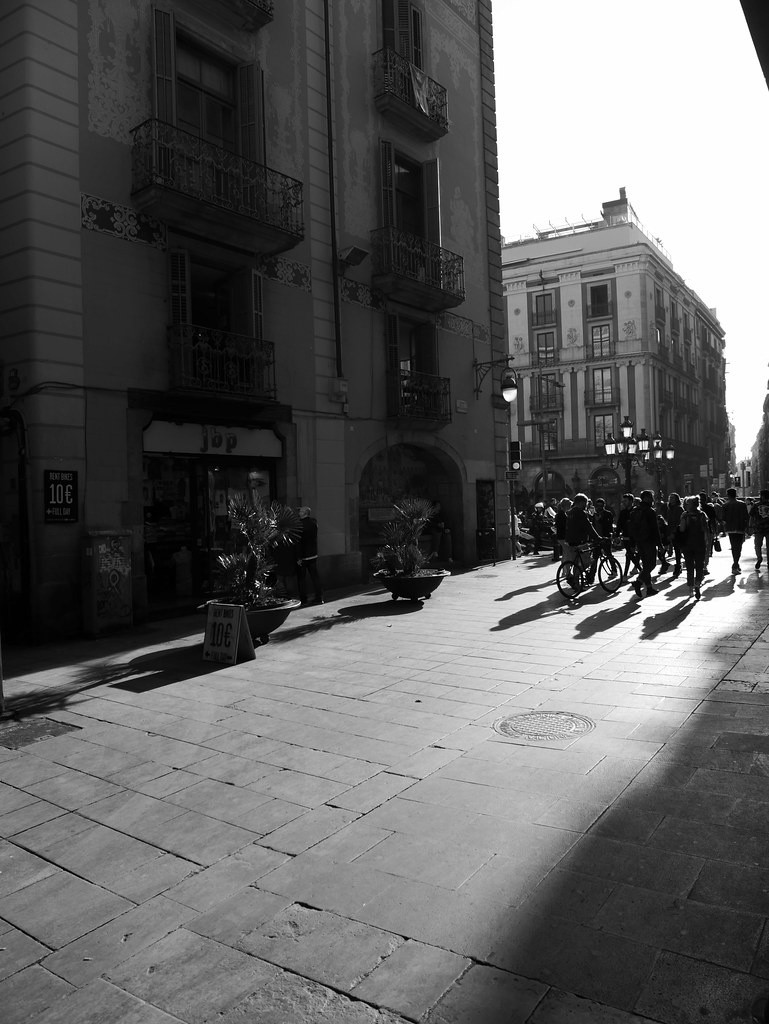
[689,587,694,598]
[630,569,638,574]
[632,581,643,597]
[647,587,659,595]
[673,564,682,575]
[738,567,741,571]
[609,571,617,579]
[721,534,726,537]
[310,599,324,605]
[756,559,762,569]
[732,569,741,575]
[665,563,670,570]
[658,568,666,574]
[567,576,586,592]
[300,599,308,606]
[695,586,701,601]
[622,576,627,582]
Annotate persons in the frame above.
[422,500,455,563]
[271,506,324,604]
[509,487,769,600]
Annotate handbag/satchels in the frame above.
[712,534,721,551]
[673,526,689,545]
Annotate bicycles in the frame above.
[556,536,623,599]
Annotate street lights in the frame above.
[644,432,675,502]
[603,415,648,494]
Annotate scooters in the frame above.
[519,517,562,556]
